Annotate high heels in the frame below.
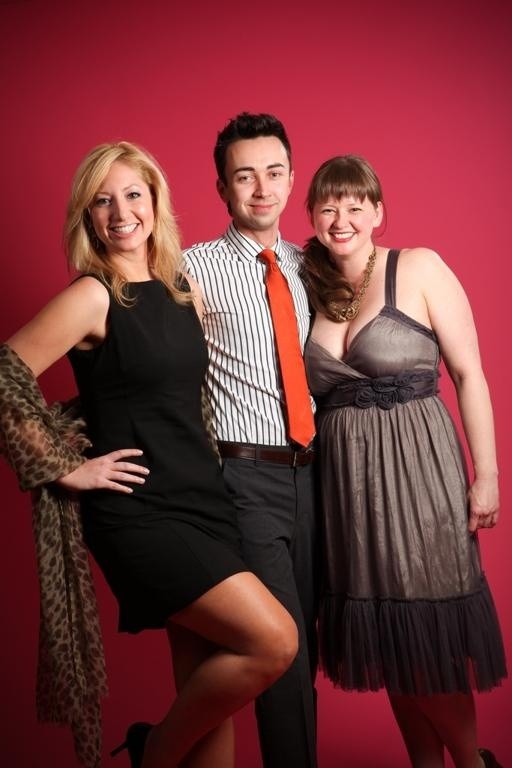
[109,721,153,768]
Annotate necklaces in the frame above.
[304,246,380,323]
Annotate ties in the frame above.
[258,249,317,448]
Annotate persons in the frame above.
[1,138,303,768]
[294,152,511,767]
[165,106,323,767]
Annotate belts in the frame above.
[217,440,318,468]
